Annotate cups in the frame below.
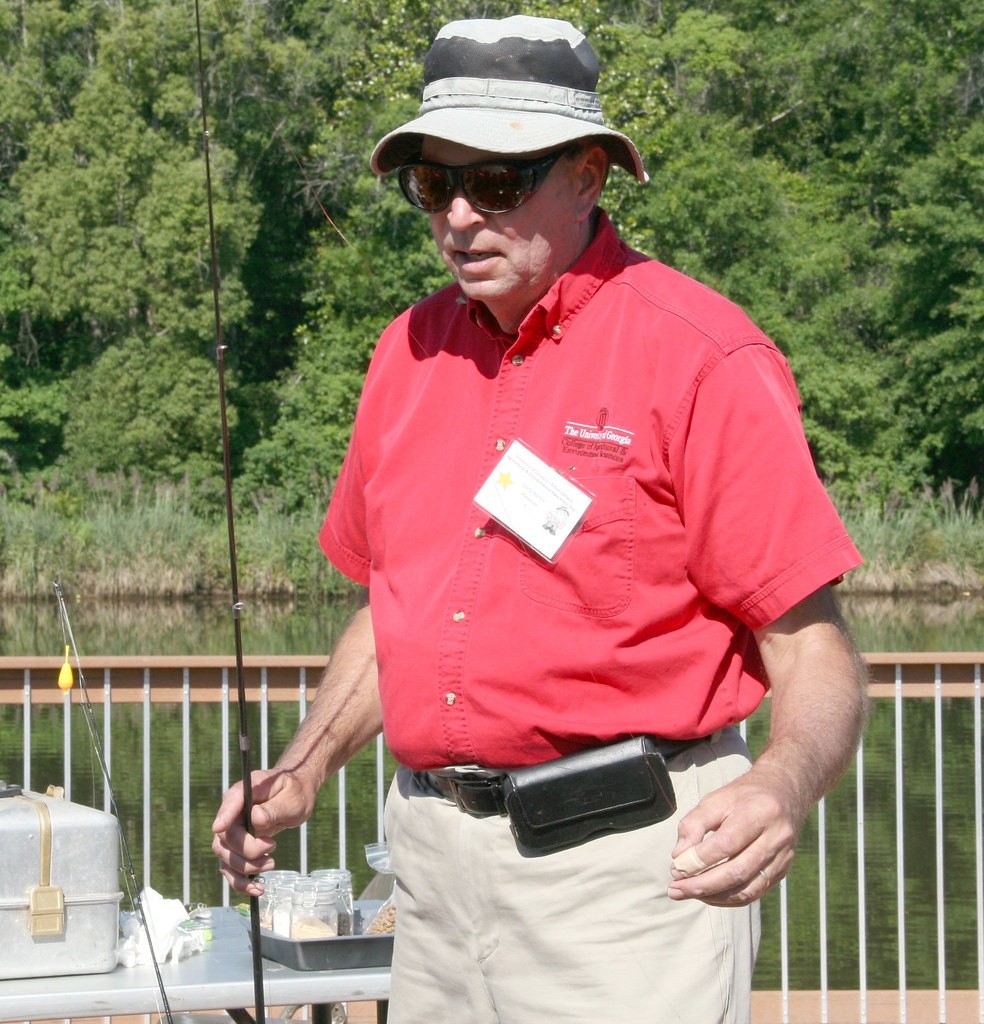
[257,868,357,939]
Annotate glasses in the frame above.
[398,144,570,215]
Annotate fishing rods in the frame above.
[49,576,175,1024]
[191,2,270,1022]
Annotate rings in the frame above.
[759,870,770,889]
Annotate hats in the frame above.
[370,15,650,182]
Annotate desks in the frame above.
[0,899,394,1024]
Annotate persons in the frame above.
[214,16,870,1024]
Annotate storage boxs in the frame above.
[239,909,396,972]
[0,779,124,980]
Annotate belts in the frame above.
[412,736,708,815]
